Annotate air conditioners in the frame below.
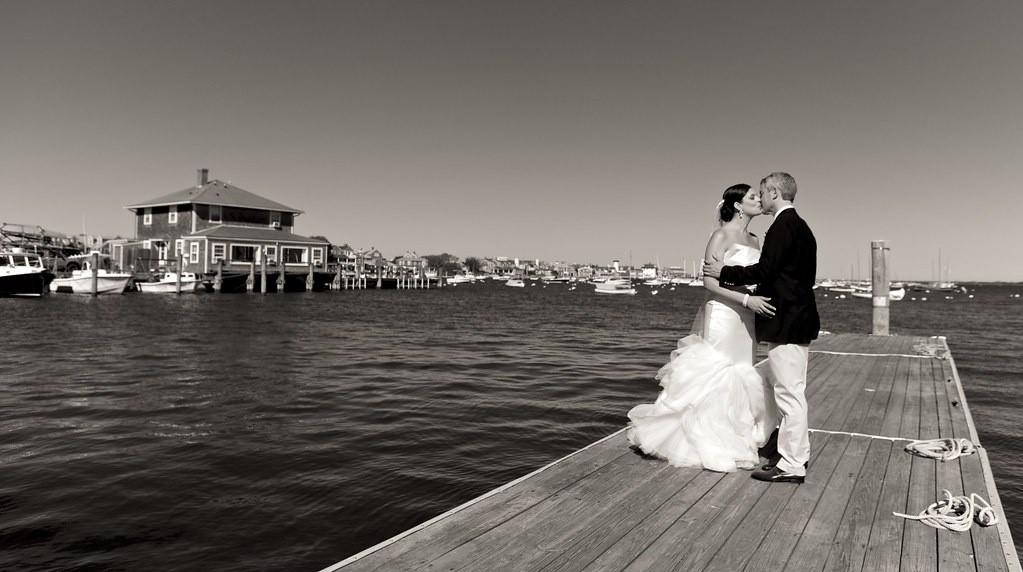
[272,222,281,228]
[267,260,275,266]
[314,260,322,266]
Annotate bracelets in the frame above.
[742,294,749,307]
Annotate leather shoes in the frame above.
[751,465,804,484]
[763,462,809,471]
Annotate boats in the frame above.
[135,271,208,291]
[504,279,525,288]
[813,247,955,300]
[529,277,539,281]
[0,247,56,296]
[476,273,511,281]
[49,250,135,295]
[446,277,472,283]
[542,249,704,294]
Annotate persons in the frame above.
[702,172,820,484]
[626,183,779,473]
